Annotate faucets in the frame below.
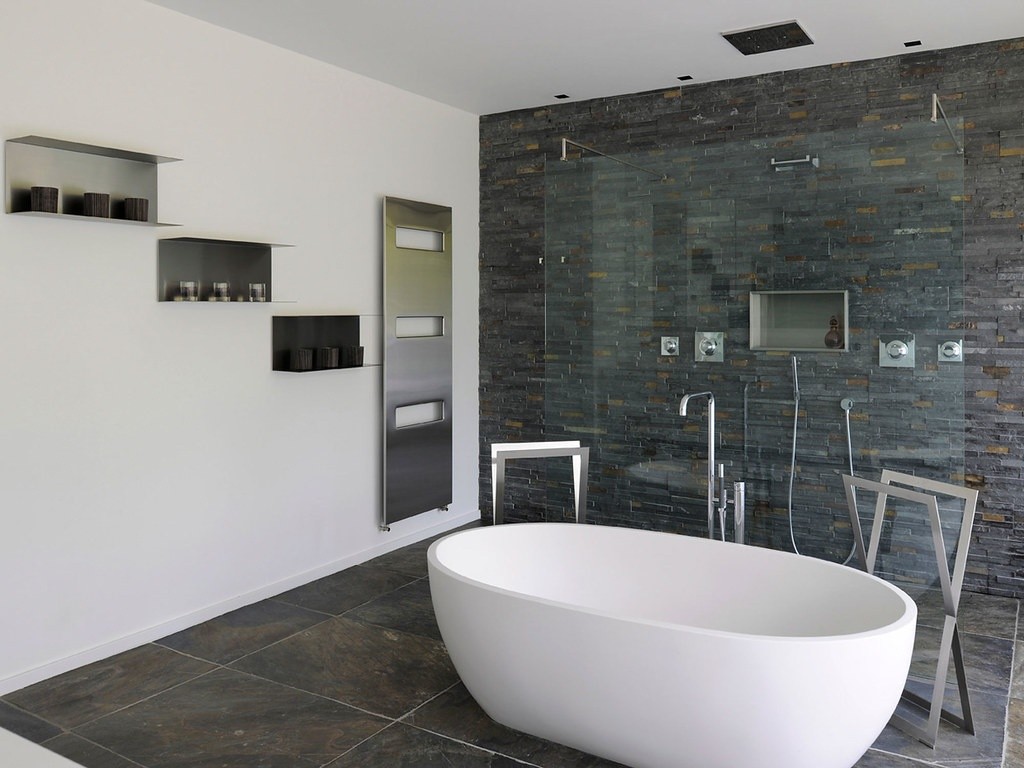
[679,390,745,546]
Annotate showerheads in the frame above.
[791,355,801,402]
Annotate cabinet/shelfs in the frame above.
[3,135,382,373]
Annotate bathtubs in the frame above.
[426,521,920,768]
